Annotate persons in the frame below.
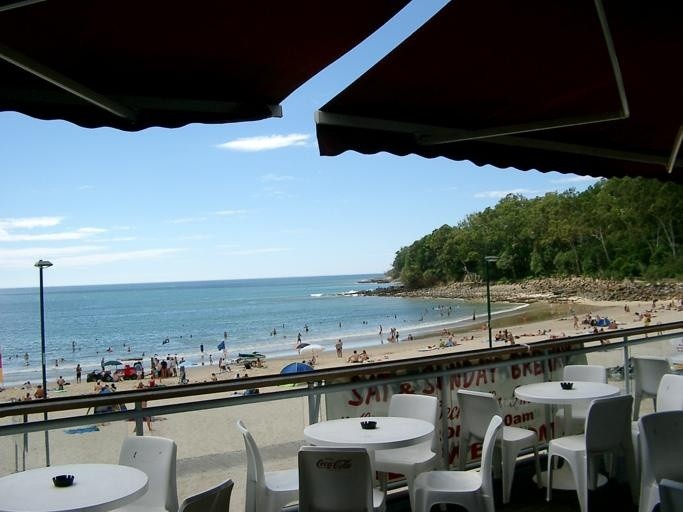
[93,344,131,370]
[624,300,682,322]
[481,324,486,330]
[378,324,413,341]
[76,364,82,383]
[133,383,153,431]
[558,313,617,345]
[472,310,477,320]
[270,323,319,365]
[495,328,551,343]
[429,328,475,350]
[644,317,651,338]
[335,339,368,363]
[72,341,76,352]
[656,320,662,336]
[142,339,190,386]
[23,352,66,401]
[86,380,128,414]
[431,304,451,317]
[200,331,265,388]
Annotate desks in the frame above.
[303,415,433,487]
[0,462,149,512]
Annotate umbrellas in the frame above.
[313,3,683,191]
[0,1,406,133]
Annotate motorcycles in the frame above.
[239,351,265,362]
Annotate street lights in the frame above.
[482,256,501,347]
[31,258,53,466]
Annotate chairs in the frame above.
[374,393,448,512]
[296,444,387,511]
[179,477,233,512]
[108,436,179,511]
[234,420,299,511]
[412,414,503,511]
[457,355,683,511]
